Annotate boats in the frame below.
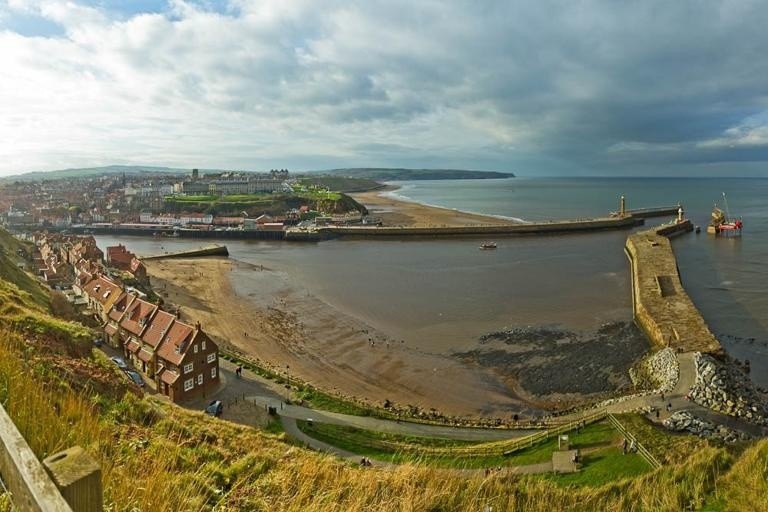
[478,240,496,251]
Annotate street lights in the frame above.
[286,364,289,402]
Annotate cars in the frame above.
[206,399,224,417]
[110,355,145,387]
